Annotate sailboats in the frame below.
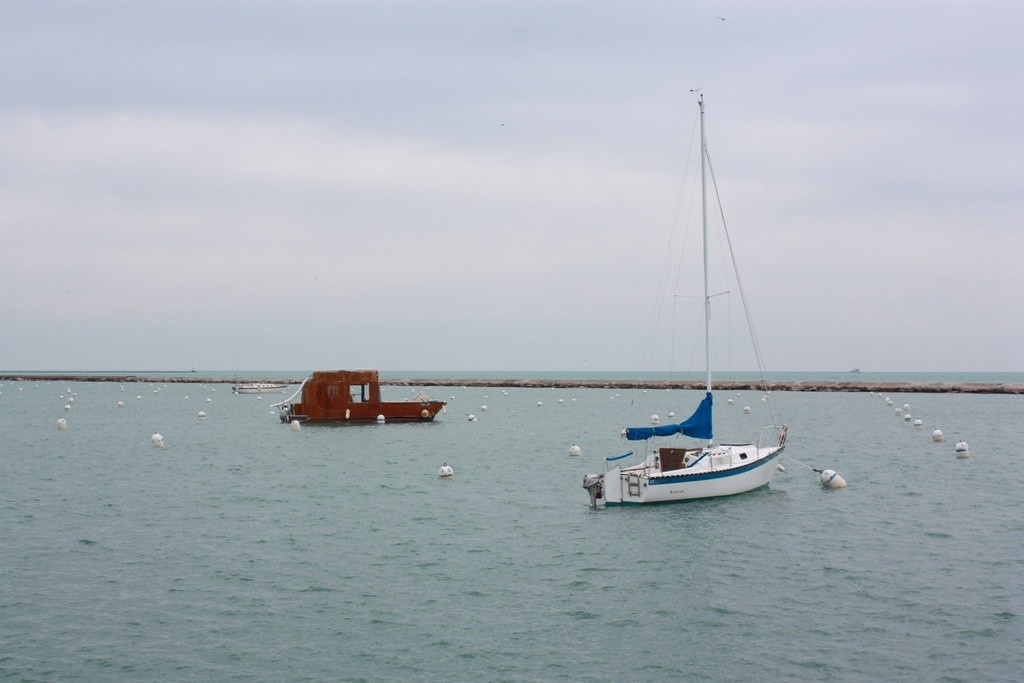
[584,89,788,508]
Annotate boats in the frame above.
[269,369,448,424]
[231,381,289,393]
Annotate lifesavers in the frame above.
[282,408,292,423]
